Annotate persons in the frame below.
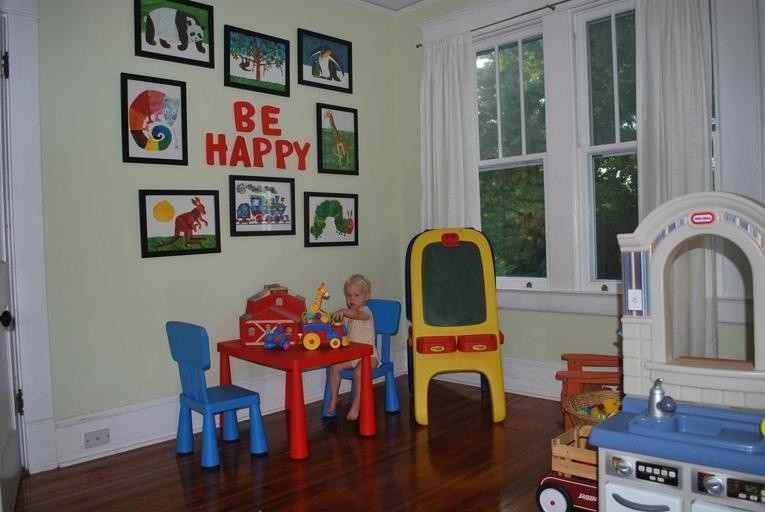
[325,275,383,423]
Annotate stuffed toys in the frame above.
[572,397,621,421]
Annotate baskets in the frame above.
[562,390,623,426]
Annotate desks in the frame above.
[215,335,380,461]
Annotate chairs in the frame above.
[321,297,402,421]
[164,318,270,470]
